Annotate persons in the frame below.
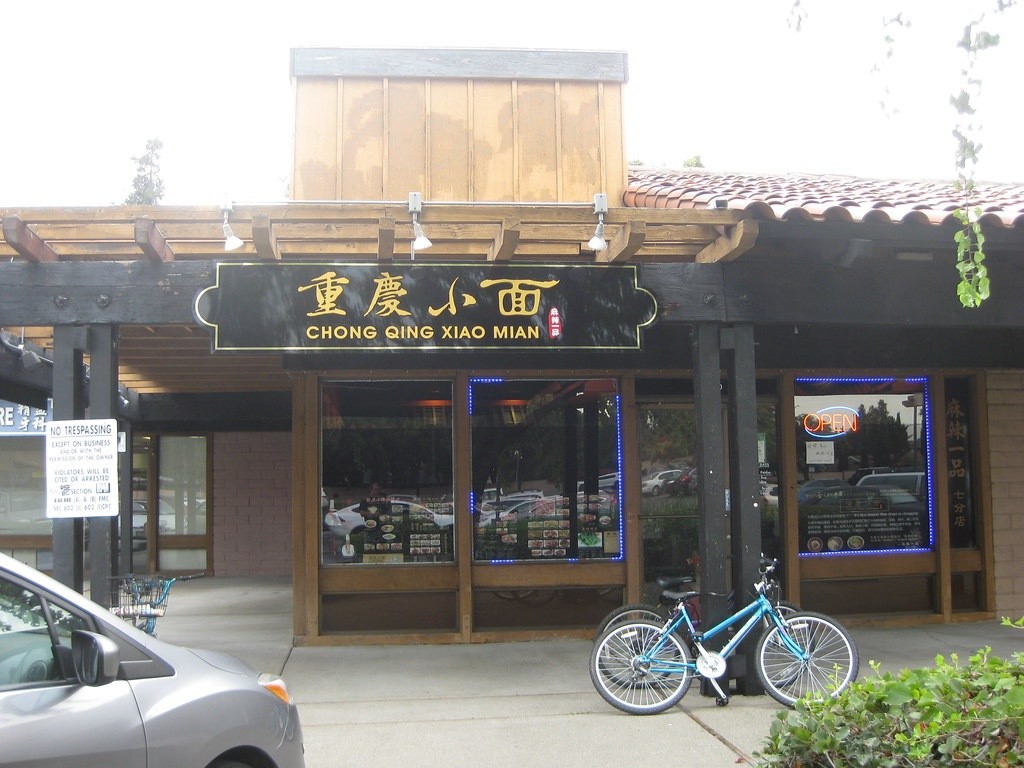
[359,483,419,554]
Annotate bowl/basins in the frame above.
[598,516,611,524]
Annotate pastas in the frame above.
[364,496,453,554]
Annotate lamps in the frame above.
[21,351,43,370]
[413,212,432,251]
[222,210,245,252]
[588,212,607,250]
[120,395,130,407]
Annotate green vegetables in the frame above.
[582,531,600,545]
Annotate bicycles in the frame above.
[590,552,859,717]
[104,570,205,640]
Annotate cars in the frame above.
[116,499,188,537]
[330,499,453,546]
[0,489,53,536]
[478,472,620,528]
[133,468,174,492]
[181,498,207,520]
[761,467,927,539]
[388,493,420,505]
[640,466,698,499]
[0,552,305,767]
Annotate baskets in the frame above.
[106,575,170,618]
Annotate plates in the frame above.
[827,536,843,551]
[806,537,823,553]
[365,514,397,539]
[576,491,616,522]
[847,535,864,550]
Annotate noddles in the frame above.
[809,537,864,551]
